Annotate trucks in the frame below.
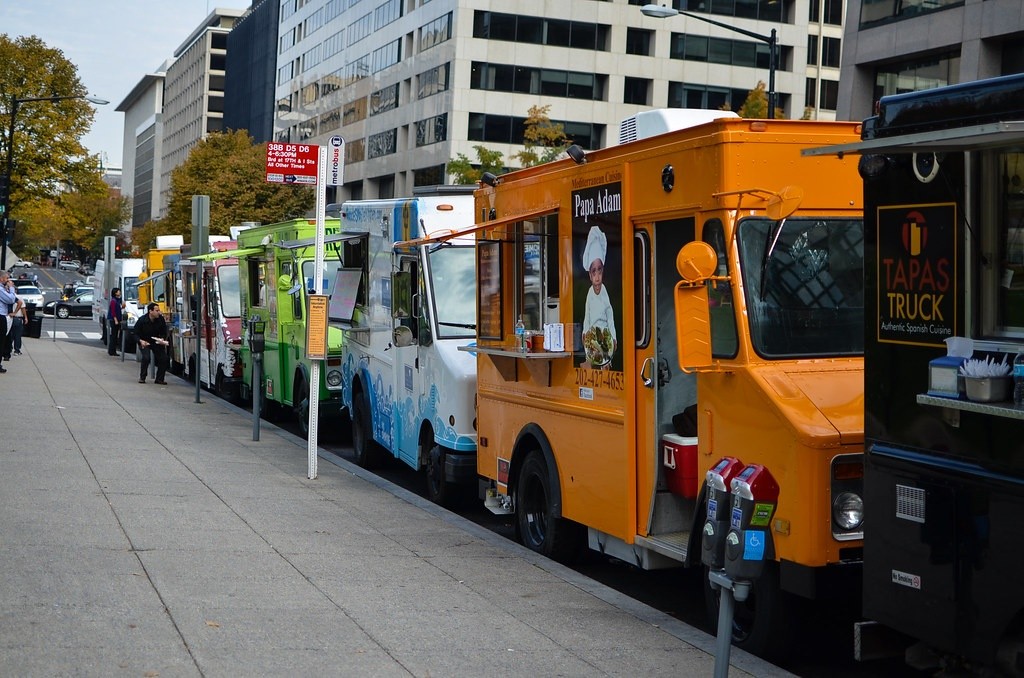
[272,182,491,505]
[393,106,865,606]
[186,218,350,440]
[133,224,243,403]
[800,67,1023,678]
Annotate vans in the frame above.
[92,257,146,353]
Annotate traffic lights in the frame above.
[116,245,121,258]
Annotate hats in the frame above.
[582,226,608,272]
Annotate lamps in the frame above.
[857,152,888,180]
[481,172,500,187]
[567,144,588,165]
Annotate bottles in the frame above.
[515,319,525,349]
[1011,347,1024,407]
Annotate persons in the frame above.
[134,303,167,385]
[108,288,126,357]
[581,227,617,360]
[1,255,54,374]
[259,278,266,307]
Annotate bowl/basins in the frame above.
[956,374,1012,402]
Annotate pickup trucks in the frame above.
[13,258,34,268]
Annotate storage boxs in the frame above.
[662,434,699,500]
[957,373,1013,402]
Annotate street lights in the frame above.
[2,94,111,272]
[640,3,776,118]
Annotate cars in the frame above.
[76,286,94,294]
[73,260,81,266]
[86,276,95,286]
[83,264,95,274]
[6,278,43,291]
[59,261,80,270]
[16,286,46,311]
[42,291,95,318]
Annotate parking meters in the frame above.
[247,314,266,440]
[119,312,129,362]
[700,456,781,677]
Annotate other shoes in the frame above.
[139,377,145,382]
[109,352,120,356]
[4,354,11,361]
[14,349,21,356]
[154,379,168,384]
[0,365,7,372]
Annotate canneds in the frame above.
[522,331,532,353]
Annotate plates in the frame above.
[584,337,614,366]
